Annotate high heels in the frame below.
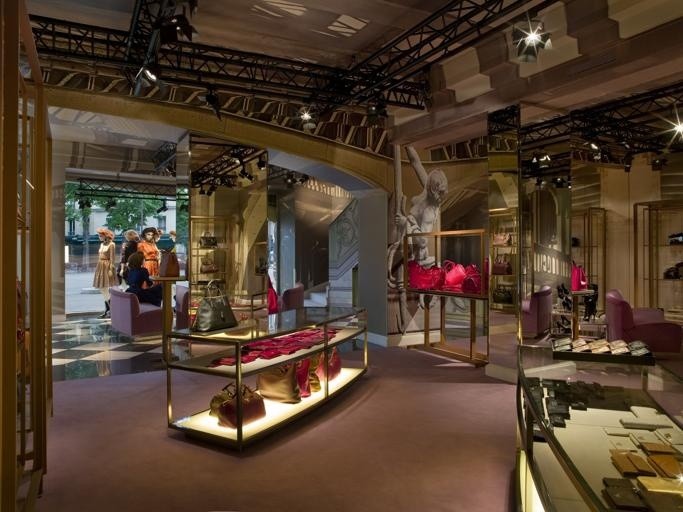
[557,285,564,300]
[561,282,568,294]
[562,301,571,313]
[561,314,572,326]
[565,297,573,304]
[555,321,567,333]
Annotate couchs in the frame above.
[281,283,304,311]
[110,288,162,336]
[521,286,552,338]
[606,290,683,353]
[176,284,190,328]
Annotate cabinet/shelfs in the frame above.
[516,337,683,512]
[404,229,489,366]
[650,208,683,315]
[488,208,518,313]
[189,216,229,326]
[165,307,368,453]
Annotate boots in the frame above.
[97,300,111,318]
[584,284,598,321]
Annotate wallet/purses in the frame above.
[648,453,683,476]
[611,449,656,479]
[620,404,673,430]
[235,329,339,362]
[572,400,587,412]
[631,430,660,448]
[607,385,623,393]
[654,425,681,446]
[522,376,603,398]
[532,429,548,441]
[604,476,632,486]
[548,400,570,418]
[636,488,682,512]
[535,399,543,419]
[211,358,237,366]
[552,418,567,427]
[634,475,680,489]
[555,336,650,356]
[606,425,629,440]
[603,486,642,509]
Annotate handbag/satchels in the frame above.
[283,359,311,399]
[159,250,180,277]
[316,347,343,381]
[258,363,301,405]
[200,231,218,249]
[196,286,238,333]
[209,386,240,417]
[492,233,512,246]
[267,275,279,314]
[308,373,320,394]
[443,261,466,292]
[219,386,267,430]
[408,260,444,291]
[492,255,514,276]
[463,264,482,294]
[493,285,513,304]
[200,262,219,274]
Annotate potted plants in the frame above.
[256,256,269,273]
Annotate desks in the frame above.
[255,273,269,305]
[560,290,595,340]
[149,276,186,363]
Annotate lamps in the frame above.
[199,189,206,194]
[85,199,91,207]
[587,140,611,164]
[227,180,232,188]
[206,94,223,120]
[257,161,266,170]
[207,190,212,196]
[551,178,566,188]
[158,14,176,51]
[285,175,296,187]
[535,178,542,186]
[652,159,667,170]
[239,170,245,178]
[365,98,378,127]
[512,27,550,61]
[143,63,160,81]
[132,81,141,97]
[247,174,254,180]
[176,13,198,42]
[157,207,168,213]
[531,153,551,168]
[106,201,116,210]
[624,151,632,172]
[78,199,83,209]
[299,175,308,185]
[180,204,188,212]
[419,79,434,108]
[210,186,217,190]
[301,107,316,130]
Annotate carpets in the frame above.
[432,331,683,417]
[18,347,516,512]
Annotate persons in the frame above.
[122,251,162,305]
[137,228,162,287]
[394,146,448,290]
[119,230,140,283]
[93,229,118,318]
